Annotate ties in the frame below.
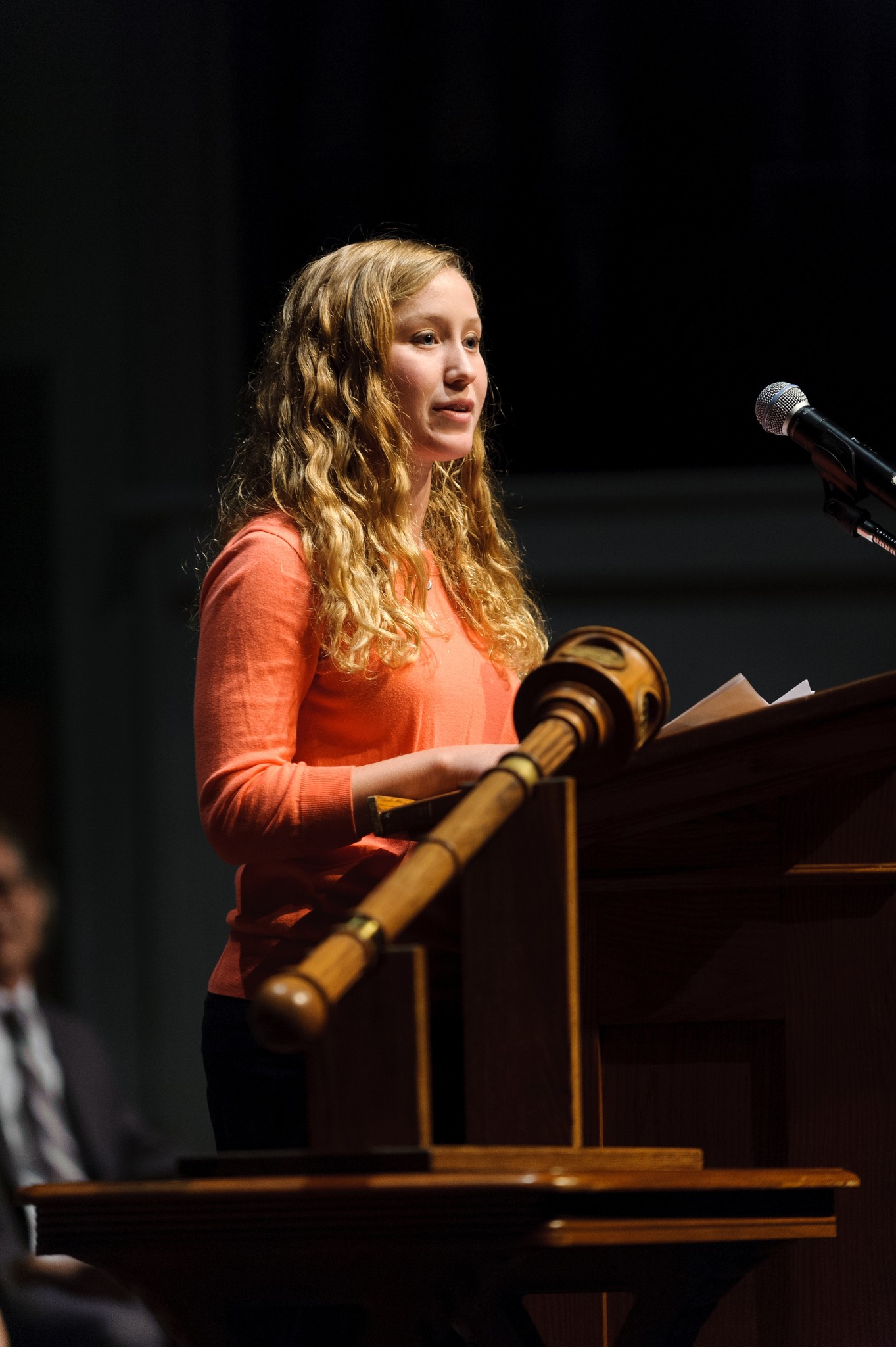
[7,1008,60,1186]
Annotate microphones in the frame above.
[752,378,896,513]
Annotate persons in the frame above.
[1,833,184,1347]
[198,238,560,1162]
